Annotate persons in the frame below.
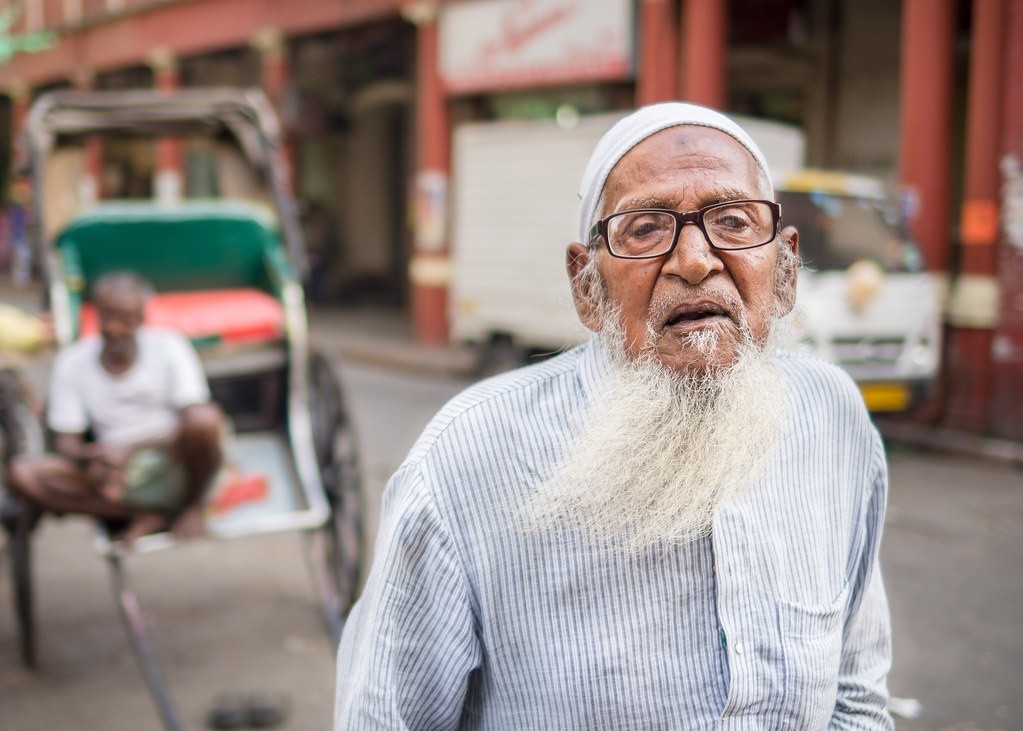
[330,100,894,730]
[10,268,229,554]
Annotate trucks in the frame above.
[447,110,942,420]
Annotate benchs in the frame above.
[79,286,284,435]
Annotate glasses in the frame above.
[588,199,783,260]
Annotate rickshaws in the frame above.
[0,89,371,731]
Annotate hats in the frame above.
[573,102,780,248]
